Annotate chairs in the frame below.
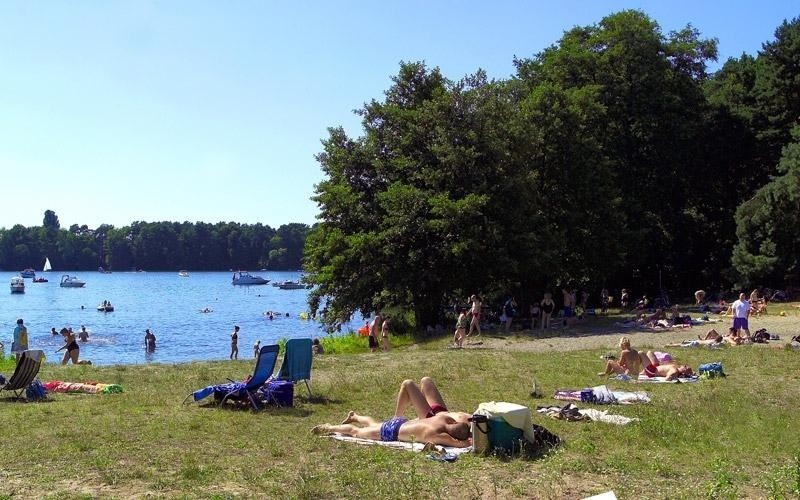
[254,338,313,404]
[181,345,280,413]
[0,350,45,403]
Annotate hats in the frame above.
[695,290,705,300]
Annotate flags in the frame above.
[43,257,52,272]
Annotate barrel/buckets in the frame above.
[487,408,523,451]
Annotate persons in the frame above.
[381,315,390,352]
[365,321,369,330]
[254,340,261,358]
[597,337,693,383]
[286,312,289,316]
[313,338,323,353]
[310,410,474,448]
[452,284,586,348]
[270,315,273,319]
[395,376,474,433]
[266,311,270,315]
[145,328,156,349]
[9,298,111,365]
[230,325,241,360]
[368,316,380,353]
[600,286,766,348]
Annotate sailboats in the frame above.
[41,257,53,272]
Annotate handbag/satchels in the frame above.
[699,362,722,377]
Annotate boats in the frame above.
[9,267,87,294]
[231,270,313,290]
[97,305,116,312]
[178,270,190,277]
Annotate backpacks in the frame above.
[26,381,45,402]
